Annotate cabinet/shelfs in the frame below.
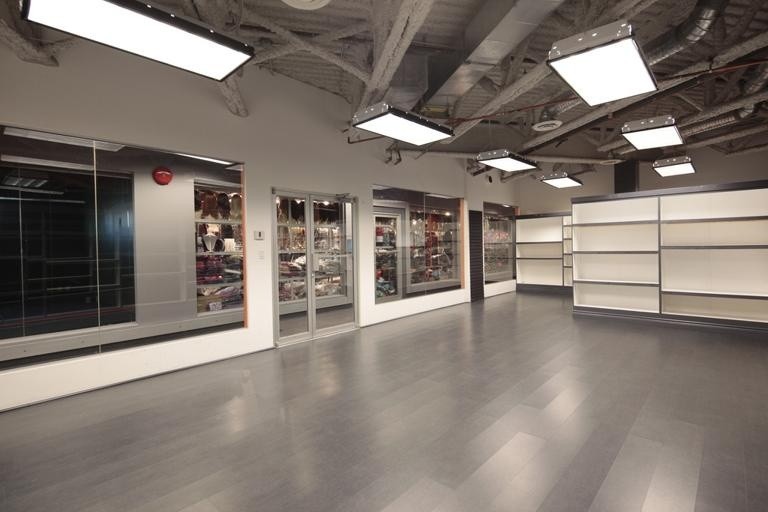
[190,184,462,327]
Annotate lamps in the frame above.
[352,88,456,149]
[545,18,696,178]
[476,107,583,188]
[17,0,253,83]
